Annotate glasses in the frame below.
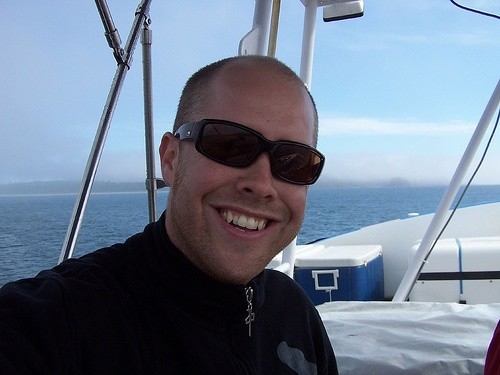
[174,119,325,186]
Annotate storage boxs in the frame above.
[294,245,385,305]
